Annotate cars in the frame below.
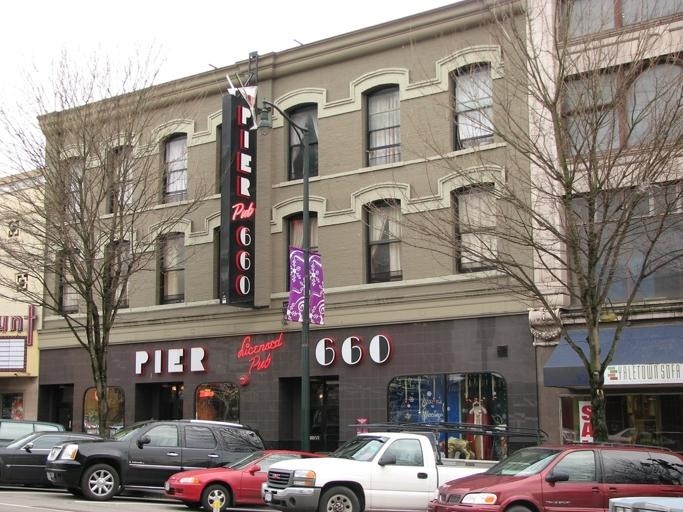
[160,452,330,508]
[0,419,107,492]
[605,426,677,446]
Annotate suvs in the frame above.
[46,420,266,502]
[426,436,682,512]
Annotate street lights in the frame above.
[255,97,319,456]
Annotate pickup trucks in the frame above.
[258,428,529,511]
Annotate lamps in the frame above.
[600,295,620,323]
